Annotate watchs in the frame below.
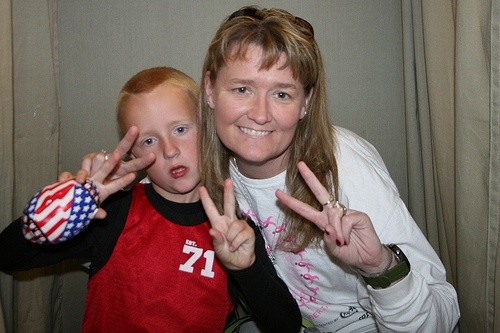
[362,243,411,288]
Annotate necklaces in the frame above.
[233,157,286,260]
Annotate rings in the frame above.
[333,202,346,215]
[323,195,334,205]
[105,152,111,160]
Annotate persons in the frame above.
[0,66,302,333]
[83,2,461,333]
